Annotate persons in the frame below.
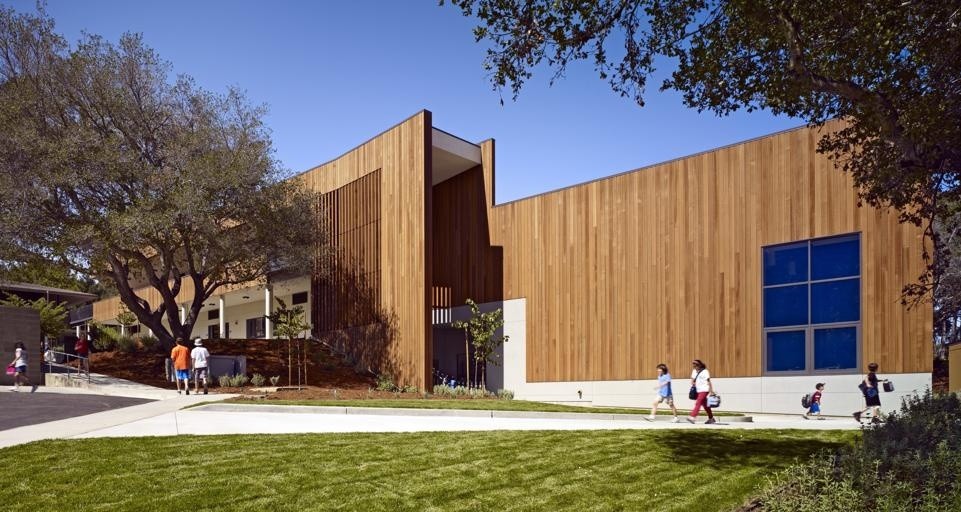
[190,337,211,395]
[73,332,93,376]
[685,358,721,425]
[642,362,682,425]
[3,339,39,394]
[848,362,890,424]
[170,336,192,395]
[801,381,827,421]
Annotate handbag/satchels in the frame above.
[883,379,895,393]
[706,393,721,408]
[6,364,17,376]
[688,386,698,400]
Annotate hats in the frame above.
[815,382,825,389]
[193,338,203,346]
[177,337,183,342]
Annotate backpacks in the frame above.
[802,394,812,408]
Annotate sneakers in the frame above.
[9,386,19,392]
[688,416,696,424]
[176,385,209,395]
[854,411,862,421]
[817,417,825,420]
[871,416,883,424]
[30,385,39,393]
[670,416,681,423]
[705,417,715,425]
[802,414,810,419]
[644,414,656,422]
